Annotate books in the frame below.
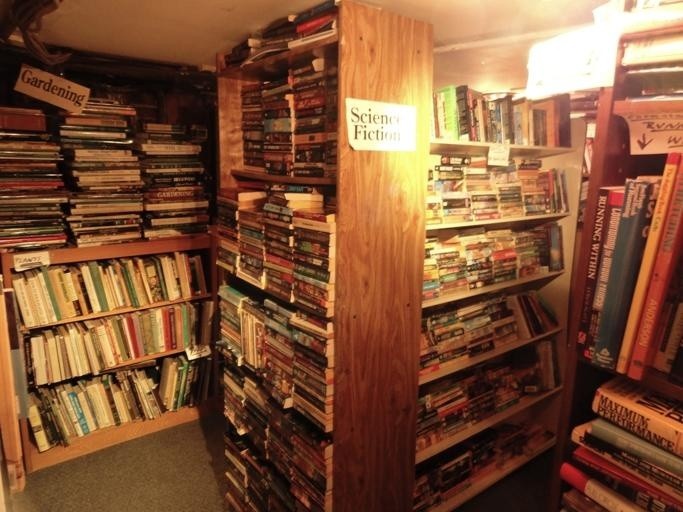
[573,152,683,386]
[431,85,571,147]
[217,183,337,317]
[241,58,338,177]
[575,138,594,230]
[26,355,211,453]
[422,221,563,300]
[23,302,213,386]
[222,367,333,512]
[415,442,516,511]
[426,153,567,224]
[571,91,599,120]
[417,338,560,448]
[420,291,559,376]
[0,94,210,253]
[223,0,338,69]
[10,251,208,329]
[216,275,333,433]
[556,378,683,512]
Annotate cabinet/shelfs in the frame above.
[549,24,681,511]
[418,86,587,511]
[0,94,216,492]
[212,2,433,507]
